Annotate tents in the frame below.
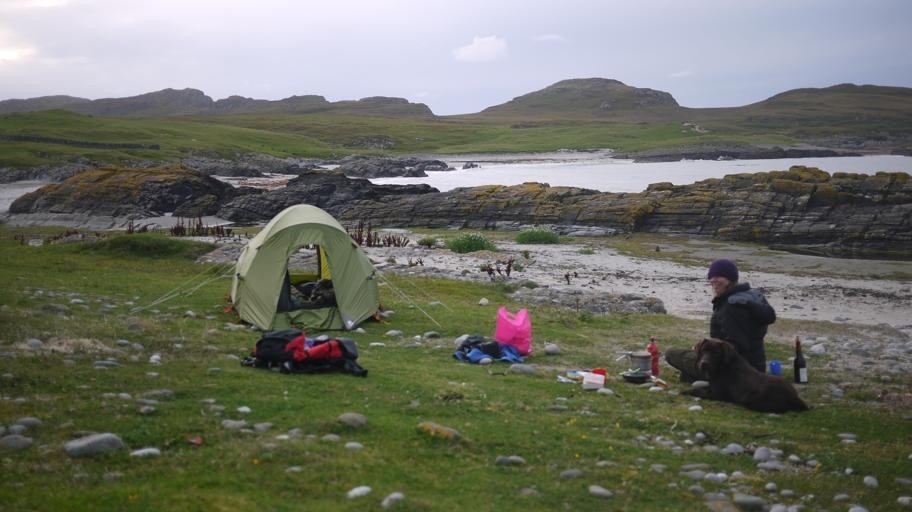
[230,204,380,332]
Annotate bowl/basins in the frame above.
[624,371,650,384]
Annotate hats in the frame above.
[707,258,739,281]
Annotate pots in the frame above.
[616,350,651,371]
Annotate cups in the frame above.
[770,362,781,377]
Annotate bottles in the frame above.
[795,337,810,383]
[645,338,661,377]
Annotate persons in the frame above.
[666,259,776,384]
